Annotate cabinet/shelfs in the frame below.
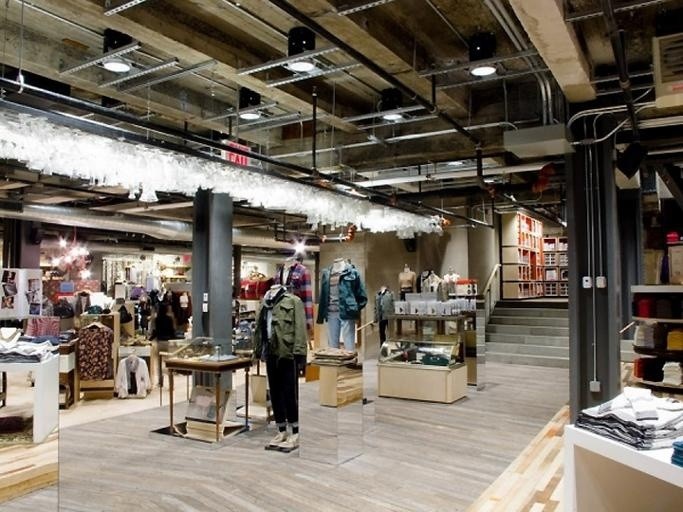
[378,332,468,404]
[501,211,545,300]
[626,284,682,401]
[320,361,363,406]
[545,238,569,297]
[44,259,265,402]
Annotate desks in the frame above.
[166,357,252,445]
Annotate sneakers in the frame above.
[269,431,288,446]
[279,433,300,448]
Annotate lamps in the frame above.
[380,86,405,120]
[101,26,133,74]
[1,76,447,244]
[238,85,262,121]
[469,32,498,77]
[287,26,317,72]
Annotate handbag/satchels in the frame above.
[53,299,74,319]
[240,272,273,300]
[87,305,102,314]
[119,305,132,323]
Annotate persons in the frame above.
[275,258,314,334]
[422,270,446,300]
[250,282,309,453]
[314,256,366,354]
[0,326,21,349]
[372,284,395,349]
[443,266,461,293]
[416,266,439,293]
[147,302,175,390]
[397,263,417,300]
[146,284,174,320]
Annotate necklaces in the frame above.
[0,327,23,344]
